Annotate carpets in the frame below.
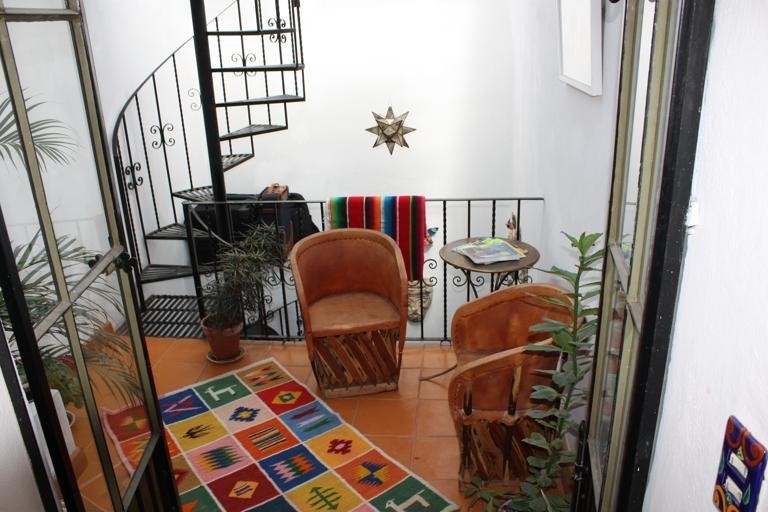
[100,357,460,511]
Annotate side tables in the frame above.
[418,237,541,382]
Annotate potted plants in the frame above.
[465,229,635,512]
[0,86,146,481]
[200,218,287,365]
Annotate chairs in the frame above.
[448,281,585,496]
[290,227,408,401]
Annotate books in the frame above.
[450,236,527,265]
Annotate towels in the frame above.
[324,195,427,282]
